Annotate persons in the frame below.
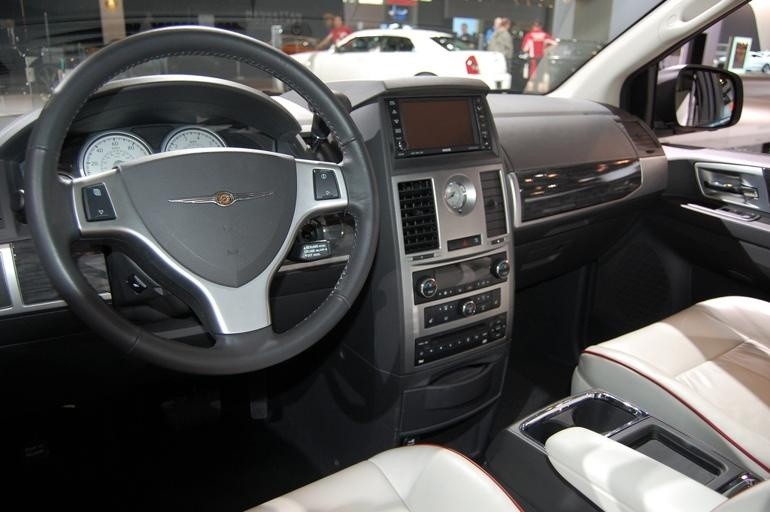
[457,17,560,94]
[317,14,352,51]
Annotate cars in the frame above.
[267,26,513,105]
[717,51,770,74]
